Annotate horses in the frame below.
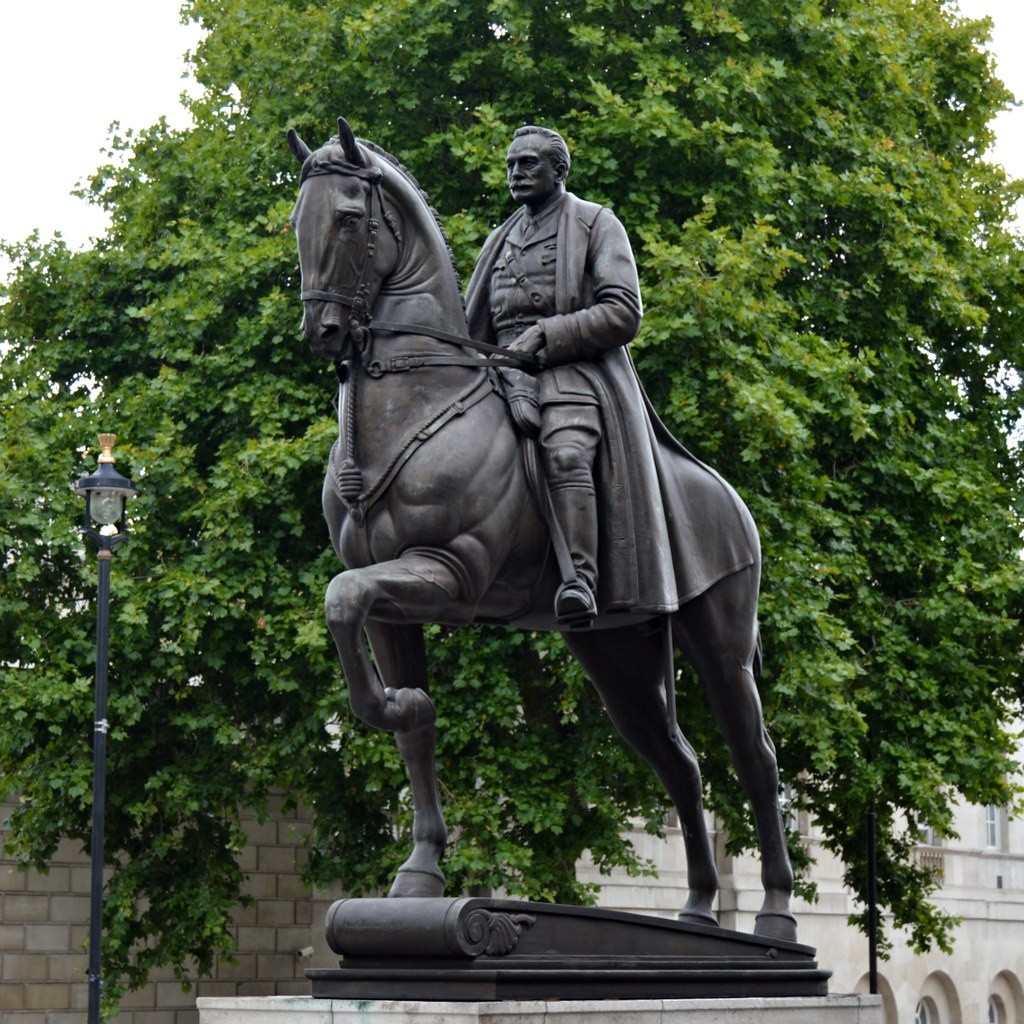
[283,115,798,944]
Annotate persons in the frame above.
[464,125,644,629]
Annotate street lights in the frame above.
[81,432,135,1024]
